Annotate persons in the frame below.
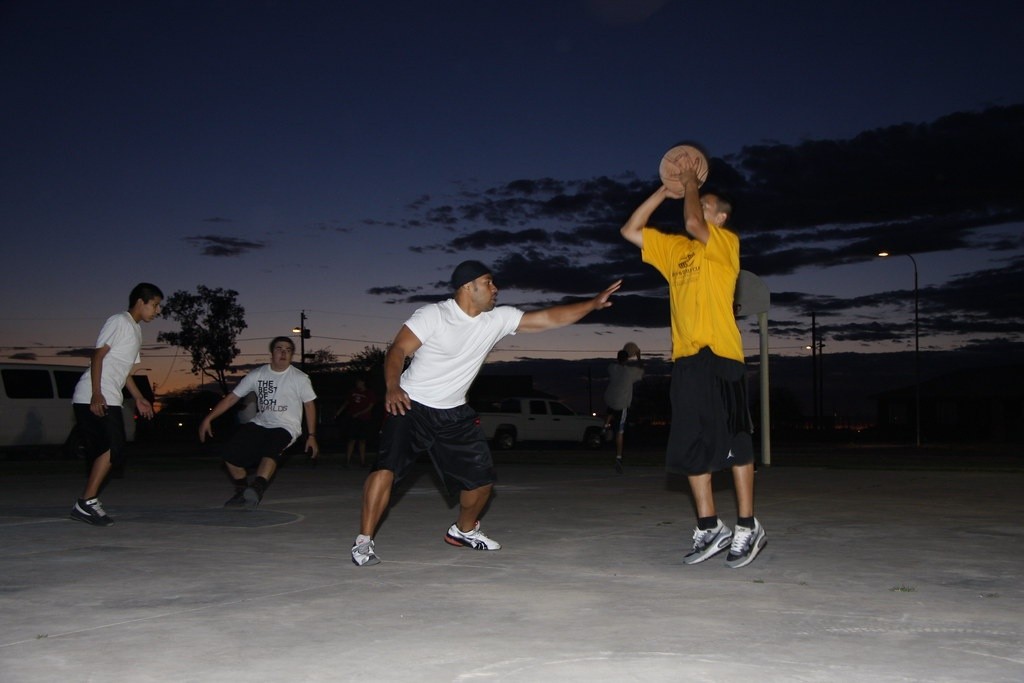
[71,282,164,528]
[199,337,318,508]
[351,261,621,566]
[601,343,645,474]
[620,145,767,569]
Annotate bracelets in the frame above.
[308,432,316,437]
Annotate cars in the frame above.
[0,362,138,465]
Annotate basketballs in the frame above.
[659,144,709,196]
[623,343,638,358]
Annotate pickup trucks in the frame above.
[476,398,612,447]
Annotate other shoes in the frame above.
[600,426,606,439]
[614,456,624,474]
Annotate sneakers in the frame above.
[726,516,767,569]
[70,496,116,527]
[680,519,733,563]
[444,520,501,551]
[226,478,267,509]
[351,533,381,567]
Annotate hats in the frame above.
[452,260,490,288]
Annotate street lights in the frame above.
[879,251,919,351]
[292,326,305,362]
